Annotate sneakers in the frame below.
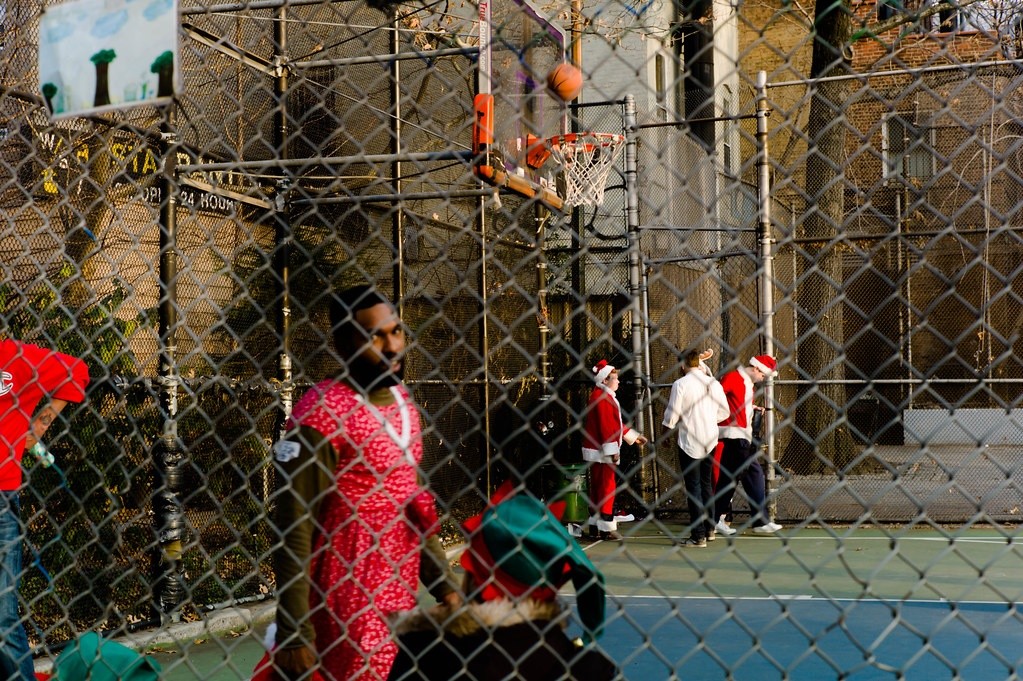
[705,531,715,541]
[589,530,623,541]
[714,521,737,535]
[754,522,783,532]
[677,537,707,548]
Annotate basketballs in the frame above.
[545,63,584,101]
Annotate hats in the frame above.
[749,354,779,377]
[698,349,714,377]
[592,359,615,383]
[458,481,606,647]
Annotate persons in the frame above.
[0,337,89,681]
[584,358,649,544]
[386,492,623,680]
[249,284,425,680]
[663,348,730,549]
[713,354,784,537]
